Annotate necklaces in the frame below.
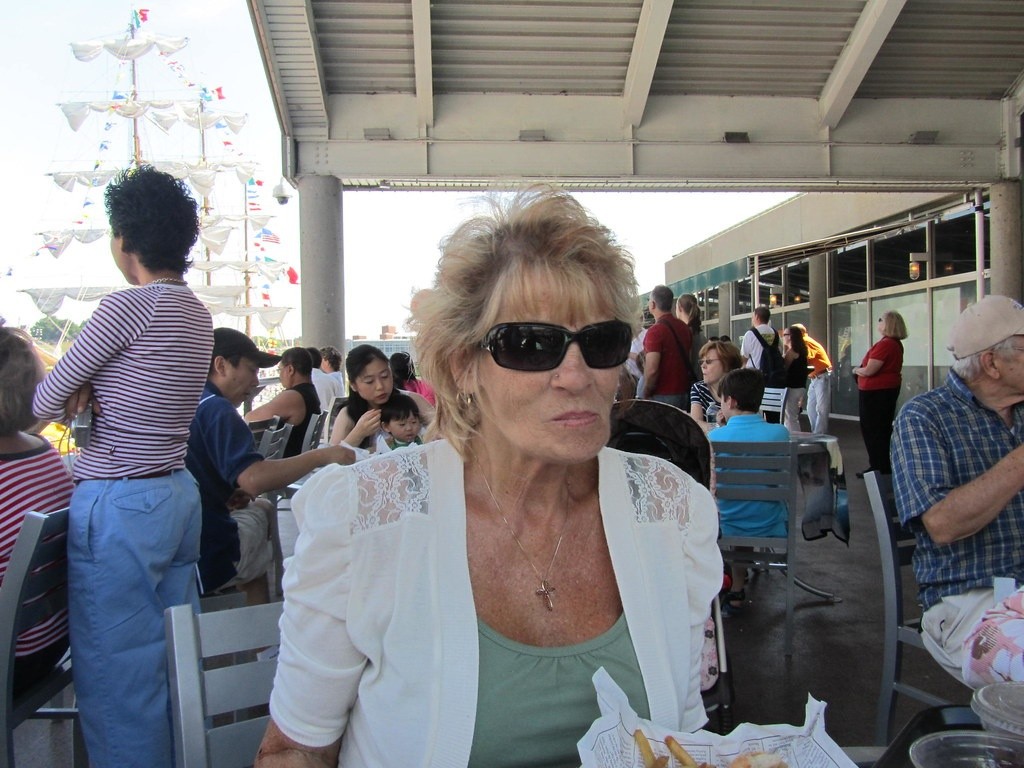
[466,443,570,612]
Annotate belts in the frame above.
[129,470,184,479]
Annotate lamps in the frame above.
[770,287,783,306]
[910,253,927,279]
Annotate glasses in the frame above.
[698,359,720,366]
[878,318,883,322]
[477,320,633,372]
[277,368,282,374]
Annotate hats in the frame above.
[946,294,1024,360]
[213,328,283,368]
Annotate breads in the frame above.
[725,752,790,768]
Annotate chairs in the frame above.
[0,386,1024,768]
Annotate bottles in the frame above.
[706,402,720,432]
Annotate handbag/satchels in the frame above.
[688,374,699,409]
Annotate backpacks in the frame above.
[747,326,789,387]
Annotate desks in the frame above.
[244,377,281,418]
[711,422,842,602]
[261,472,312,597]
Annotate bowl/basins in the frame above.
[970,680,1024,730]
[909,731,1024,768]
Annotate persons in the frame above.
[251,180,726,768]
[704,368,790,607]
[391,352,436,409]
[30,160,201,768]
[615,293,731,399]
[793,323,832,434]
[245,347,320,458]
[690,341,741,432]
[853,310,906,477]
[0,326,76,704]
[321,347,343,411]
[304,347,333,440]
[742,306,782,389]
[889,295,1024,692]
[374,394,422,456]
[781,328,807,432]
[328,345,438,462]
[639,285,693,411]
[186,328,357,593]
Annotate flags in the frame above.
[261,227,281,243]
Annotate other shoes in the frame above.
[727,593,741,607]
[855,466,875,478]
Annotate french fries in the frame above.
[635,729,715,768]
